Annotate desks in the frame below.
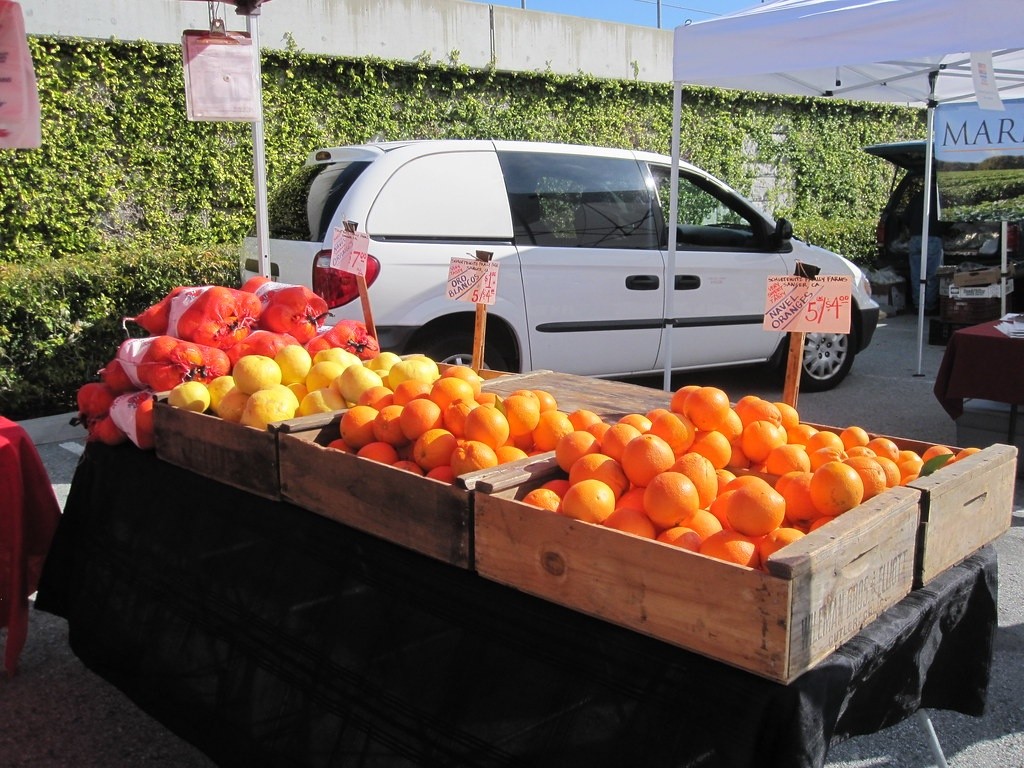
[934,312,1024,447]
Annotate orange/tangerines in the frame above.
[78,279,982,574]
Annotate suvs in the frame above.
[243,138,882,394]
[863,139,1023,302]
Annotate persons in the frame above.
[906,178,956,315]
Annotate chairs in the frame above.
[574,183,629,247]
[501,176,560,249]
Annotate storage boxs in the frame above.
[153,348,1017,687]
[863,236,1018,346]
[955,398,1024,480]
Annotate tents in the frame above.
[663,0,1024,392]
